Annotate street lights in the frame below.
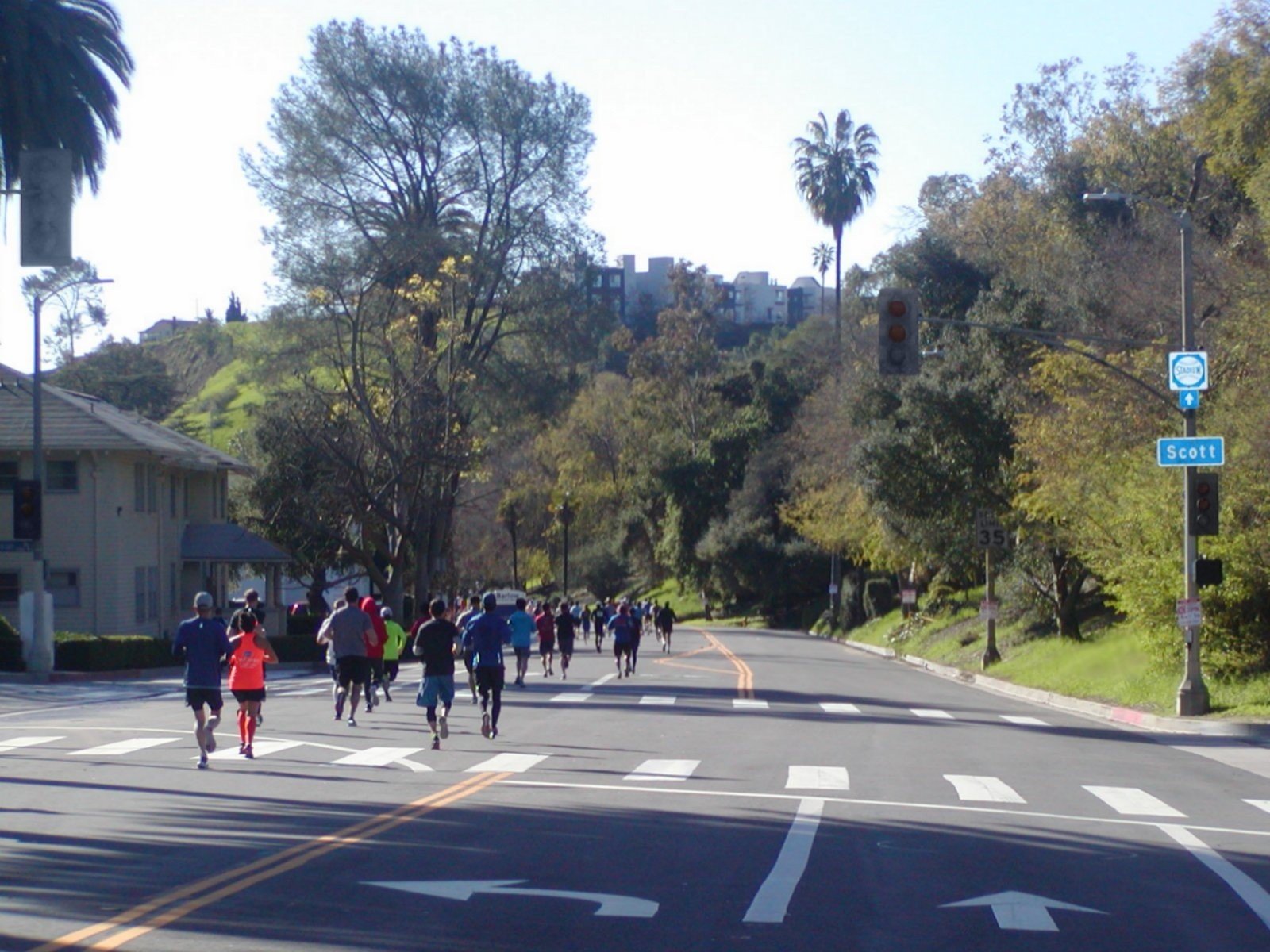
[1081,188,1213,720]
[29,277,117,686]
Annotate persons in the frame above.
[411,597,462,751]
[629,607,645,674]
[461,593,512,739]
[554,602,578,679]
[359,596,389,713]
[226,588,267,726]
[172,592,234,769]
[379,605,407,703]
[507,597,538,688]
[227,612,278,760]
[535,602,556,678]
[606,604,632,679]
[526,596,679,655]
[321,587,379,726]
[407,596,471,640]
[317,599,347,703]
[454,596,484,705]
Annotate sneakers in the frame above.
[239,741,245,754]
[481,712,490,738]
[632,668,635,673]
[348,717,355,724]
[573,628,671,654]
[203,726,216,753]
[543,673,547,677]
[628,665,631,672]
[519,680,526,687]
[617,673,621,679]
[383,674,389,689]
[386,696,392,702]
[197,761,209,769]
[365,708,371,712]
[548,668,553,675]
[371,686,379,706]
[255,715,262,726]
[432,733,439,749]
[472,698,477,704]
[561,674,566,680]
[624,668,629,677]
[515,675,521,685]
[246,743,253,758]
[333,711,341,720]
[488,728,498,739]
[563,656,568,668]
[440,717,448,739]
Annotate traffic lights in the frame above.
[876,283,922,371]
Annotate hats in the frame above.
[380,606,392,618]
[194,592,214,611]
